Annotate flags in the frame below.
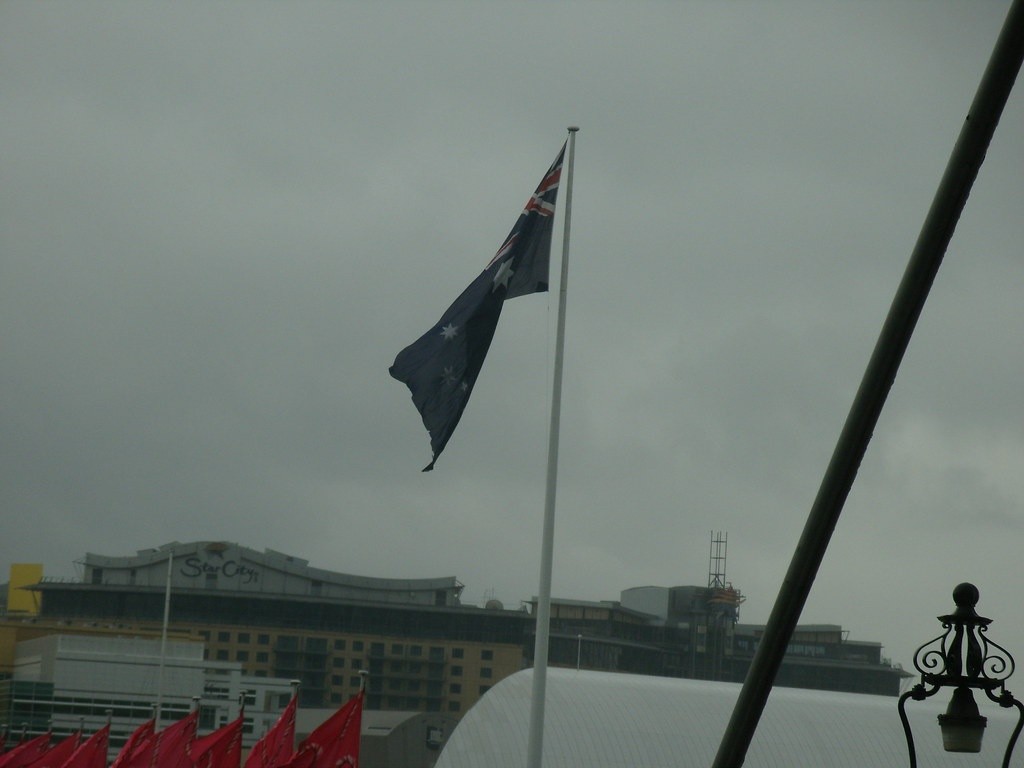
[0,684,366,768]
[386,135,569,473]
